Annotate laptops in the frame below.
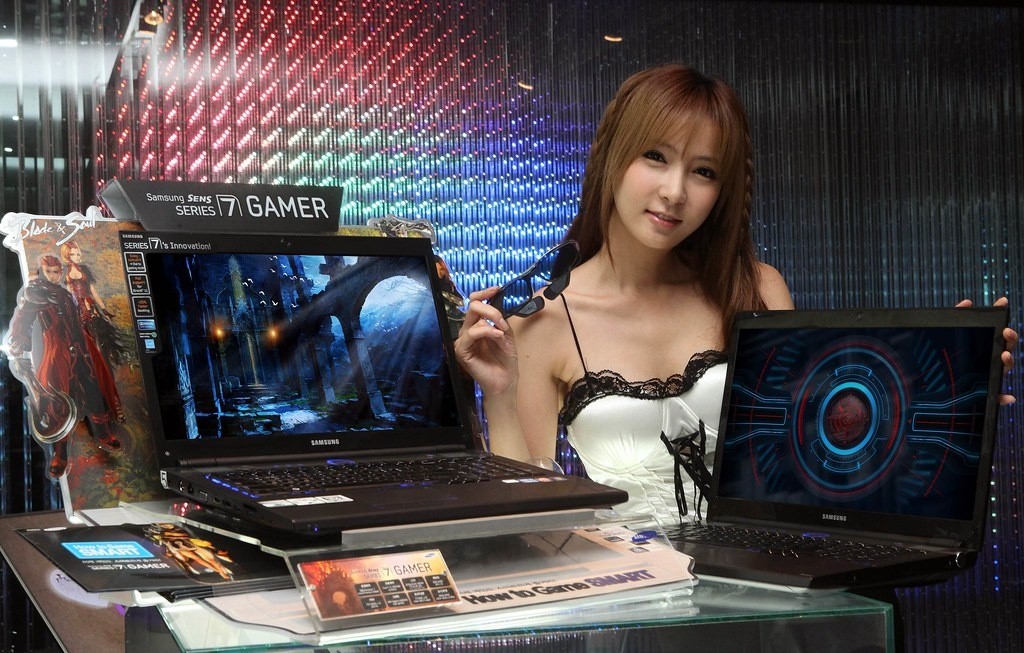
[117,227,630,538]
[632,306,1012,588]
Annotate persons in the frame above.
[455,64,1019,529]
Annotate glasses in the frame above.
[486,240,581,319]
